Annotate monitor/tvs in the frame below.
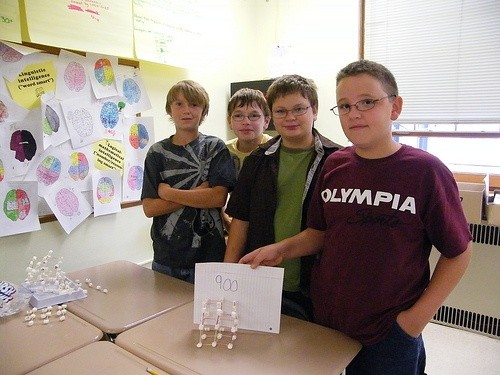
[231,80,276,130]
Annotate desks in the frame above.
[0,260,363,375]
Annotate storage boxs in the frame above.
[457,182,485,224]
[487,195,500,226]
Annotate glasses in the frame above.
[231,110,266,121]
[330,95,397,116]
[272,103,314,119]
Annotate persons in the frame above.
[238,59,474,375]
[141,79,234,286]
[222,74,348,332]
[221,88,275,234]
